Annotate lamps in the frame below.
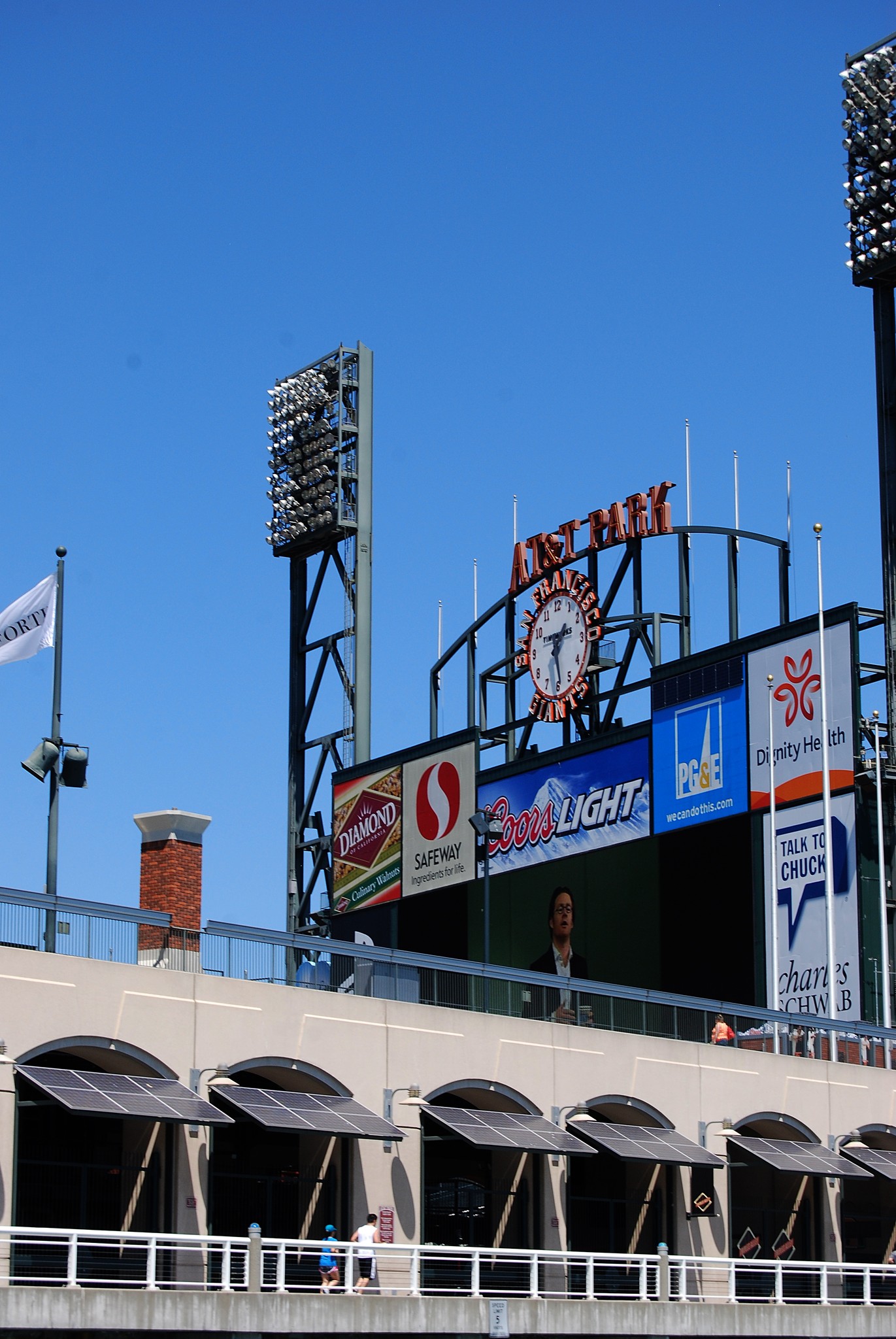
[551,1100,597,1161]
[699,1116,741,1150]
[826,1129,870,1184]
[384,1083,430,1147]
[188,1062,239,1131]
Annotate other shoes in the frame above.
[353,1289,357,1294]
[323,1288,329,1294]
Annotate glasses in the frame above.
[554,905,572,914]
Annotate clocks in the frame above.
[526,591,590,700]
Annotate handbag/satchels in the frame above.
[727,1026,735,1040]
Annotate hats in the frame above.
[325,1225,337,1232]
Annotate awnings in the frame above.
[14,1064,235,1128]
[726,1136,873,1180]
[208,1083,408,1142]
[567,1120,728,1169]
[840,1146,896,1179]
[420,1106,597,1155]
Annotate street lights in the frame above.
[837,33,895,755]
[466,808,503,1014]
[265,343,377,998]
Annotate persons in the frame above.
[807,1028,816,1057]
[319,1225,340,1294]
[793,1026,804,1055]
[519,886,596,1025]
[888,1251,896,1305]
[711,1015,727,1046]
[350,1214,382,1293]
[860,1036,870,1065]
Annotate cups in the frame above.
[579,1005,592,1026]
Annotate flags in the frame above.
[0,573,55,664]
